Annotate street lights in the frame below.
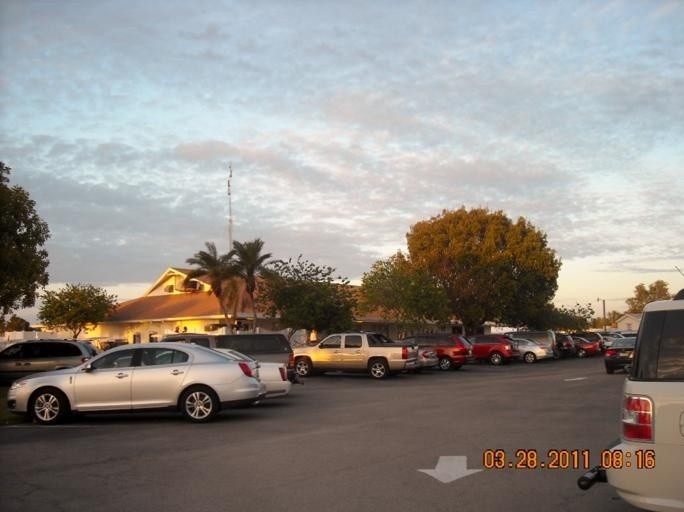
[595,296,606,331]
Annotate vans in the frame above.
[577,288,683,512]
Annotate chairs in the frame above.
[144,350,157,365]
